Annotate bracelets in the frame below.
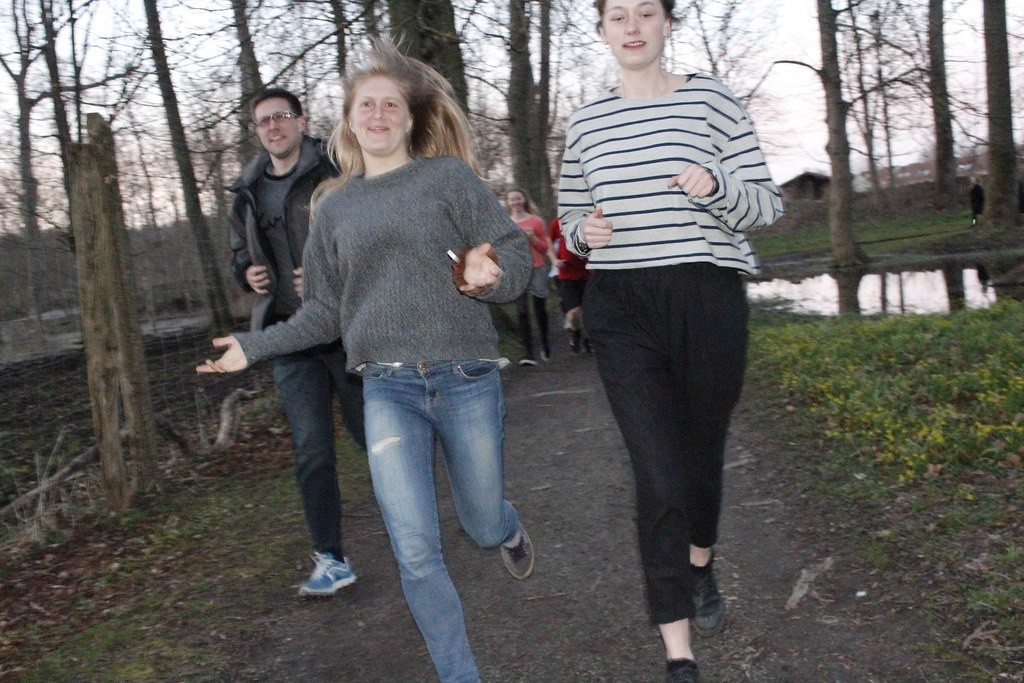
[451,243,500,297]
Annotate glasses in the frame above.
[254,110,298,127]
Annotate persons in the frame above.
[967,175,985,228]
[545,0,787,683]
[196,38,553,682]
[977,265,990,293]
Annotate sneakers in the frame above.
[499,500,535,580]
[298,551,358,599]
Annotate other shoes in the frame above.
[570,350,581,357]
[519,354,538,367]
[665,659,699,683]
[582,347,595,354]
[539,346,552,361]
[688,550,725,638]
